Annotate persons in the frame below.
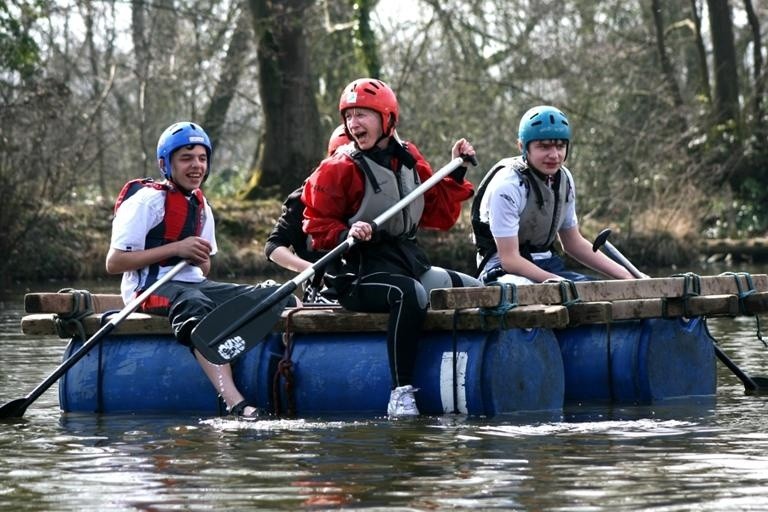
[300,78,481,418]
[104,121,303,422]
[264,123,354,307]
[469,104,636,285]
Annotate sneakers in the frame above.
[387,383,420,417]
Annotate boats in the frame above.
[22,273,764,415]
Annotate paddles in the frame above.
[593,228,768,397]
[192,154,474,365]
[0,259,191,418]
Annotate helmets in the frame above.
[157,121,213,180]
[338,77,400,140]
[328,124,351,157]
[518,105,571,161]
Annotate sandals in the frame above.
[229,399,271,421]
